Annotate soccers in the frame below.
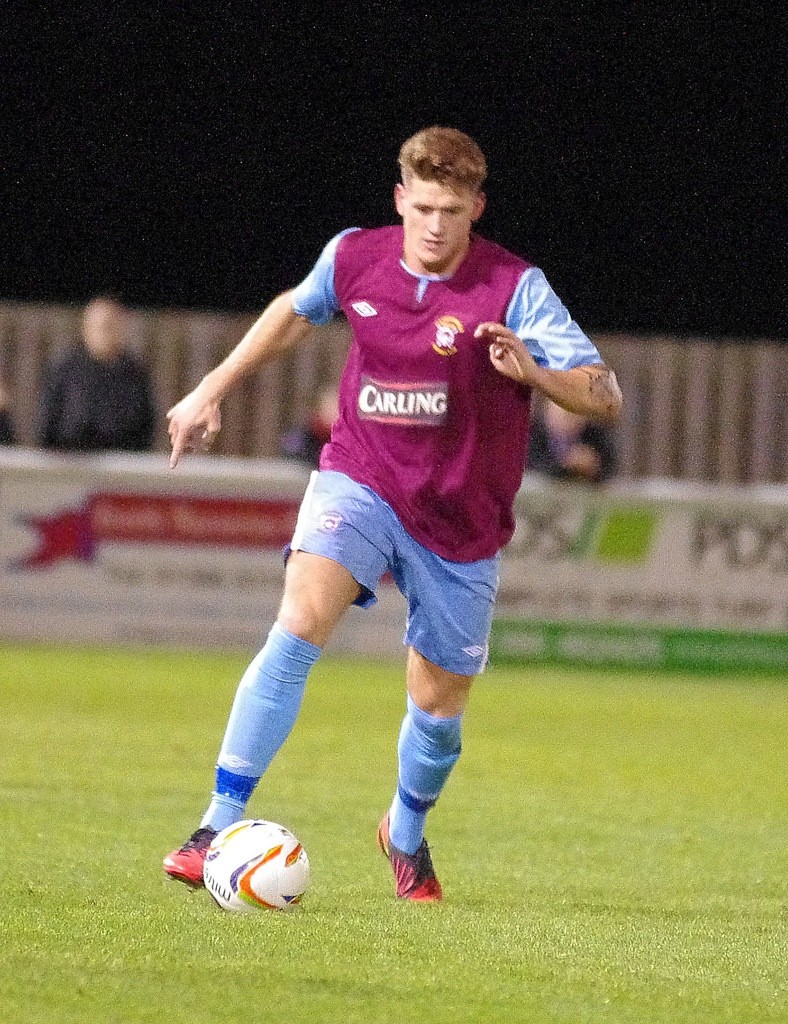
[203,818,312,916]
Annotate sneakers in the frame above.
[162,825,219,888]
[377,812,441,901]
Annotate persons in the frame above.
[35,299,157,454]
[277,375,345,471]
[165,126,623,902]
[523,389,619,488]
